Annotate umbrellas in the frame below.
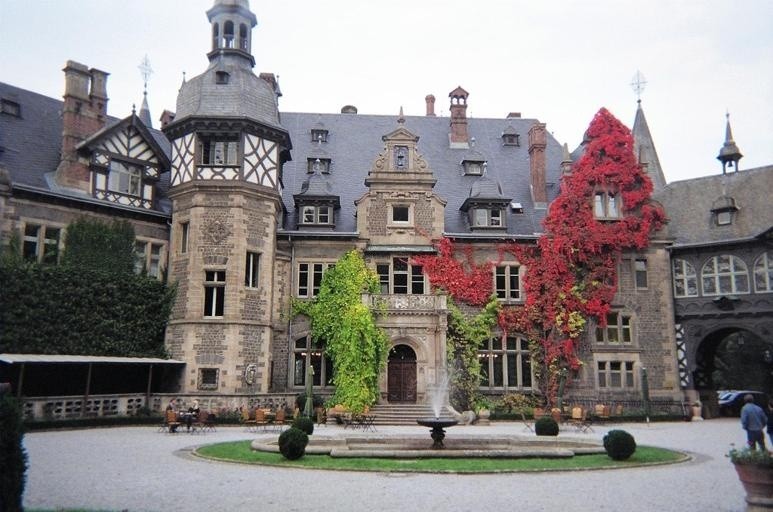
[641,367,651,424]
[557,367,567,409]
[303,365,315,416]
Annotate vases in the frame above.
[734,462,772,511]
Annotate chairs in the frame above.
[519,403,629,433]
[157,403,379,434]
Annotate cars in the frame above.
[716,389,766,418]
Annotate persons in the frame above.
[186,399,201,432]
[740,393,768,449]
[166,399,179,433]
[766,399,773,443]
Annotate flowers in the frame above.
[726,441,773,468]
